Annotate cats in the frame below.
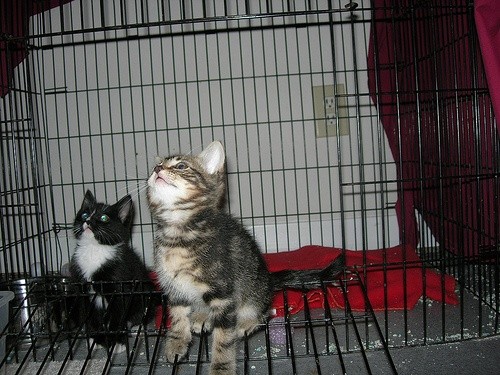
[114,141,344,375]
[63,189,161,354]
